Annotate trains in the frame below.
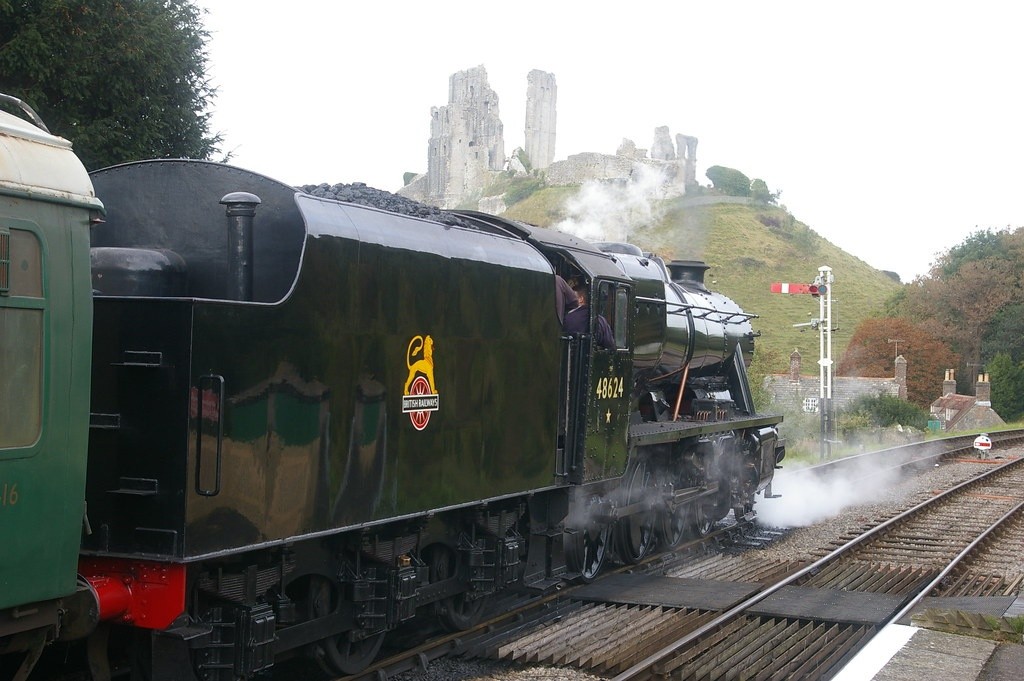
[1,91,787,681]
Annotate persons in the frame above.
[556,276,614,350]
